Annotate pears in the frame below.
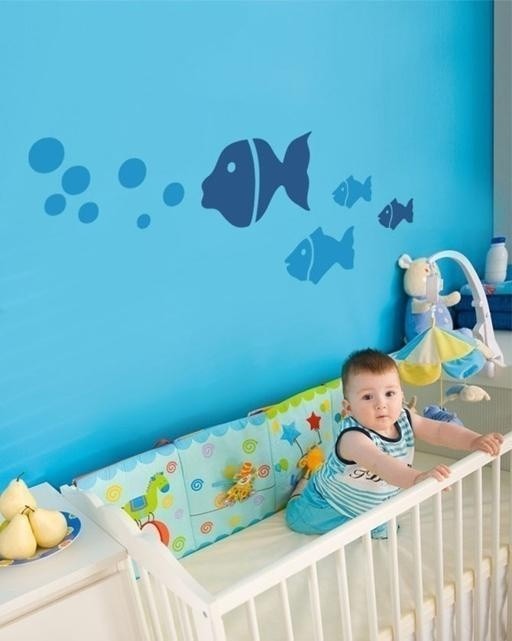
[1,472,36,521]
[24,504,67,549]
[0,507,36,559]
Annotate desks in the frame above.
[2,481,149,639]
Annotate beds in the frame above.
[64,349,512,639]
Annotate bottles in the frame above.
[483,236,508,284]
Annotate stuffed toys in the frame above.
[386,249,506,425]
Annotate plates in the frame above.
[0,510,78,569]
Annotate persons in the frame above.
[284,349,506,540]
